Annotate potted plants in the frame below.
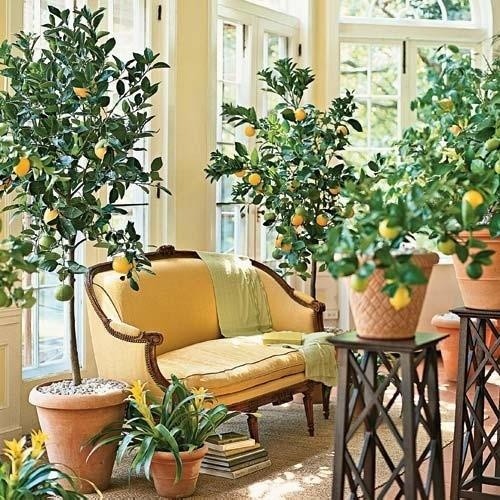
[82,375,239,498]
[204,44,499,338]
[0,0,175,496]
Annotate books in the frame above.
[263,332,302,345]
[199,432,272,480]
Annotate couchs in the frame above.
[81,245,335,442]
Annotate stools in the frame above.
[318,306,500,499]
